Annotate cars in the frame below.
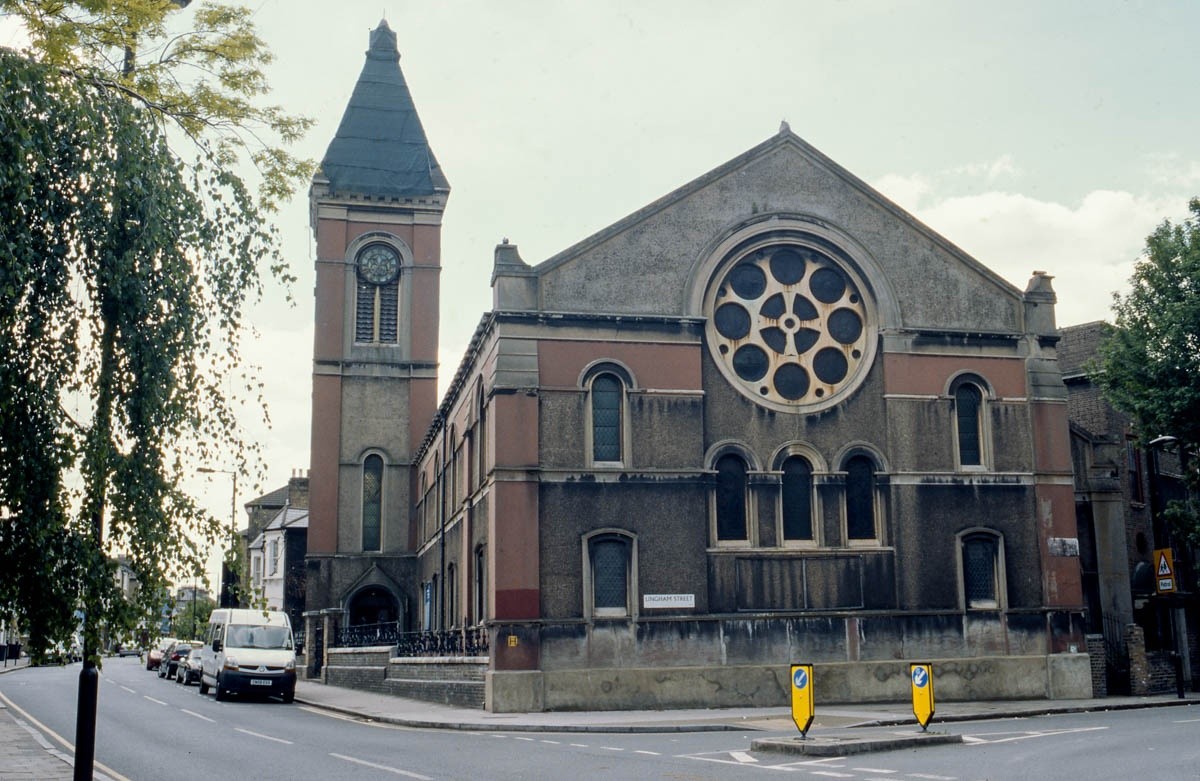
[41,635,83,663]
[175,649,204,686]
[119,640,144,657]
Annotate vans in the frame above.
[146,636,178,672]
[198,607,306,704]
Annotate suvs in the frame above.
[158,640,204,680]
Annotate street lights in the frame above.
[1148,434,1186,700]
[197,467,237,607]
